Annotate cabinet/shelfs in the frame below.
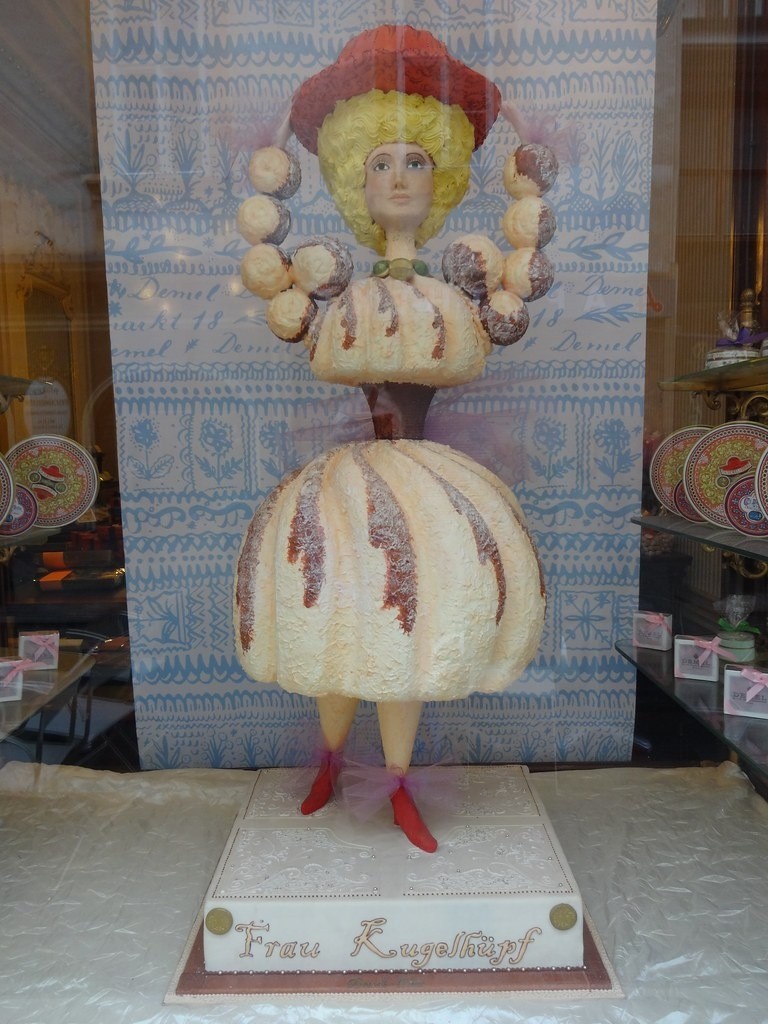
[615,355,768,780]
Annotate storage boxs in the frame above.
[19,630,60,671]
[633,611,672,651]
[674,635,719,682]
[0,656,25,702]
[723,663,768,721]
[33,525,123,592]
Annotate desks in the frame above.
[0,577,129,645]
[0,653,98,765]
